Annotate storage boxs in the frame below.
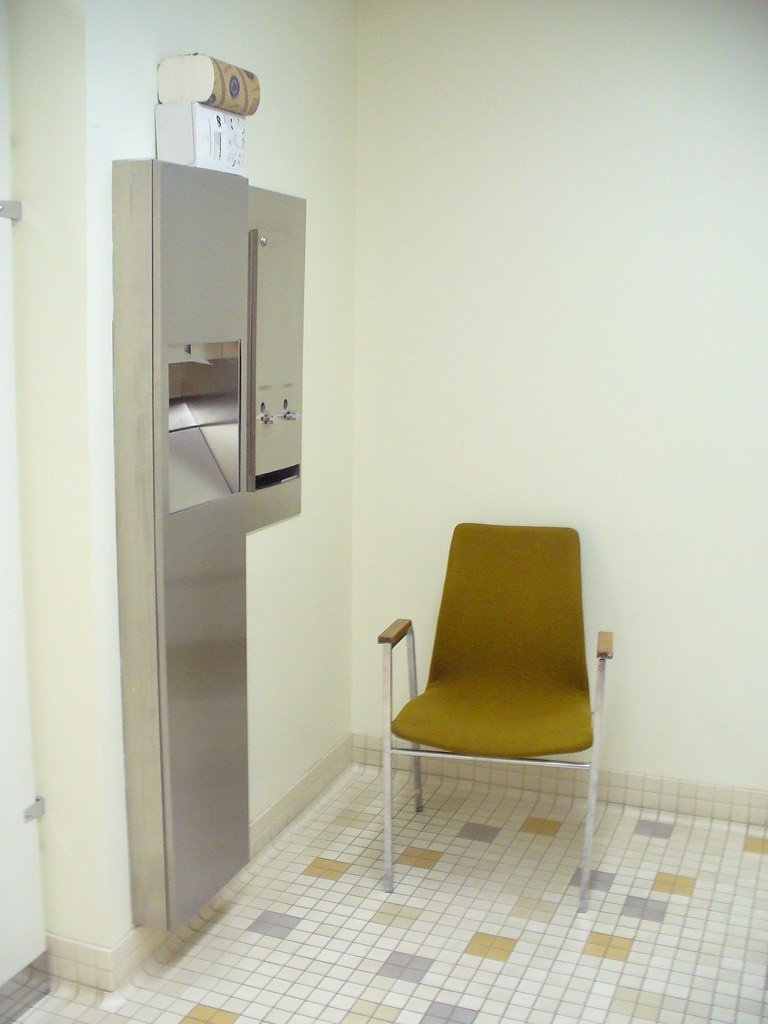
[155,101,247,177]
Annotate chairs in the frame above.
[376,523,614,912]
